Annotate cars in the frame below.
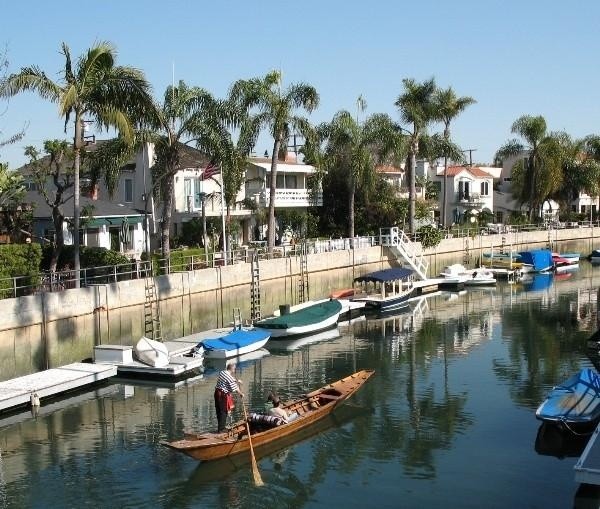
[481,223,511,235]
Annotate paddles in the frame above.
[239,383,264,487]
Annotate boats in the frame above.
[440,249,580,285]
[157,367,376,461]
[353,268,417,315]
[535,367,600,425]
[195,266,350,359]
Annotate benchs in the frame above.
[248,413,286,433]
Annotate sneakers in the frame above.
[218,428,230,433]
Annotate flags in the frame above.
[199,154,221,182]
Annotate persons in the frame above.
[213,361,242,433]
[273,449,290,469]
[263,394,274,414]
[268,396,299,423]
